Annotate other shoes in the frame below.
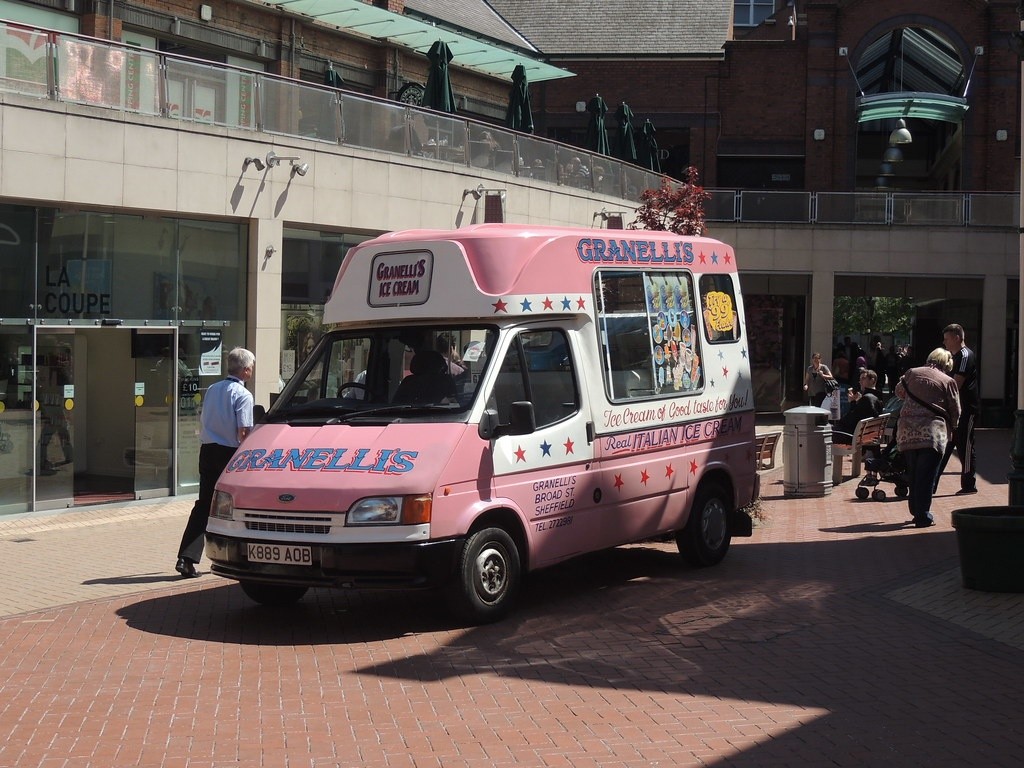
[861,478,878,486]
[41,468,57,475]
[956,488,978,494]
[66,457,73,462]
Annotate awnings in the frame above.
[715,135,807,159]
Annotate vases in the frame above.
[951,504,1024,594]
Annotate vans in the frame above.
[204,222,760,625]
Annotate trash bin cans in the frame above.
[783,406,833,498]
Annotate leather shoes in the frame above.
[176,559,203,578]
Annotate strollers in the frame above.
[855,439,908,501]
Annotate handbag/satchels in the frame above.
[822,364,841,397]
[876,348,886,370]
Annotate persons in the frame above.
[156,347,190,390]
[37,340,76,475]
[803,352,835,409]
[426,132,449,159]
[933,324,980,495]
[343,349,403,404]
[175,345,256,580]
[478,130,502,172]
[435,330,468,376]
[851,356,868,390]
[835,368,884,447]
[531,152,606,194]
[833,329,915,395]
[627,172,638,201]
[856,442,884,486]
[896,347,962,530]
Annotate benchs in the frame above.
[822,412,892,484]
[754,432,782,470]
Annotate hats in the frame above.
[857,357,865,363]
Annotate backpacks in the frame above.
[886,353,896,368]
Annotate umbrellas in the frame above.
[421,37,457,160]
[591,92,612,168]
[644,117,662,173]
[313,63,347,142]
[505,63,535,176]
[616,101,638,164]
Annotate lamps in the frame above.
[245,152,626,221]
[888,29,913,144]
[872,117,904,188]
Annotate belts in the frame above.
[42,404,62,407]
